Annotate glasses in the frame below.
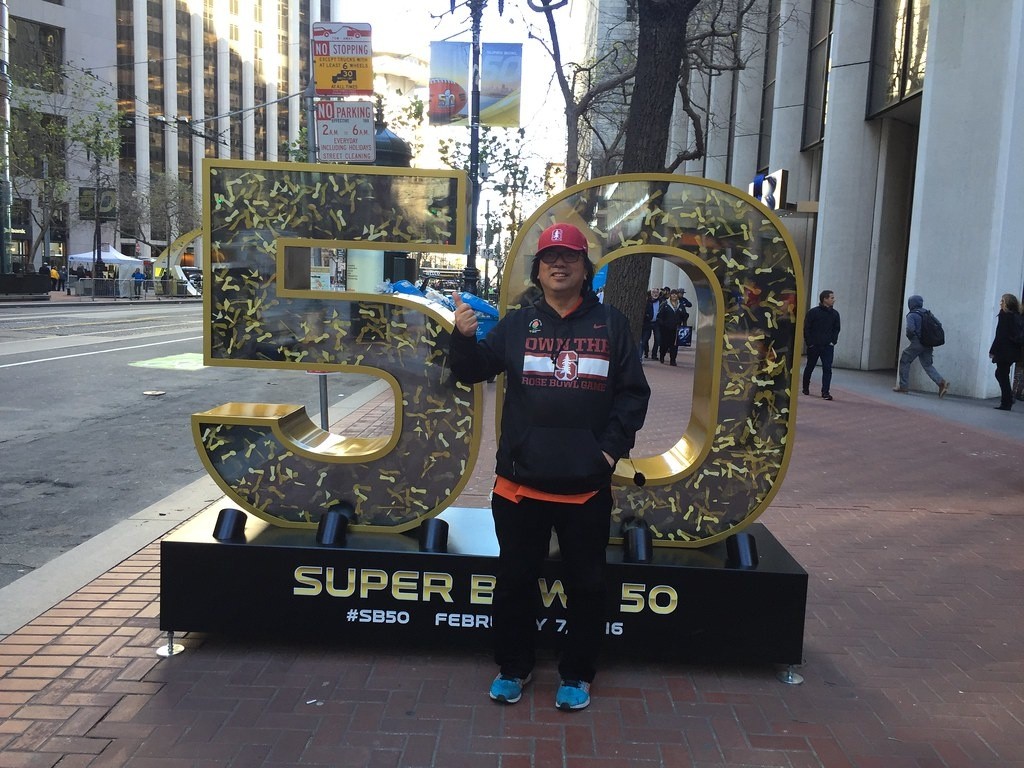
[540,250,579,263]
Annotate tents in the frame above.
[67,243,144,298]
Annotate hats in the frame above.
[668,289,679,294]
[660,287,671,292]
[677,288,686,293]
[538,223,588,253]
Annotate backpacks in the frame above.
[906,311,945,347]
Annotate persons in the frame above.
[596,283,693,365]
[892,295,950,398]
[448,223,651,711]
[802,290,840,400]
[31,259,202,300]
[989,294,1024,410]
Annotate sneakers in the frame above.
[491,670,533,704]
[802,389,809,395]
[892,385,909,393]
[555,676,591,712]
[937,379,951,398]
[821,393,833,401]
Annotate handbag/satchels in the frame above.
[676,325,692,346]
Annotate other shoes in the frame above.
[644,354,648,358]
[660,353,665,363]
[651,355,658,360]
[993,400,1012,411]
[670,356,677,366]
